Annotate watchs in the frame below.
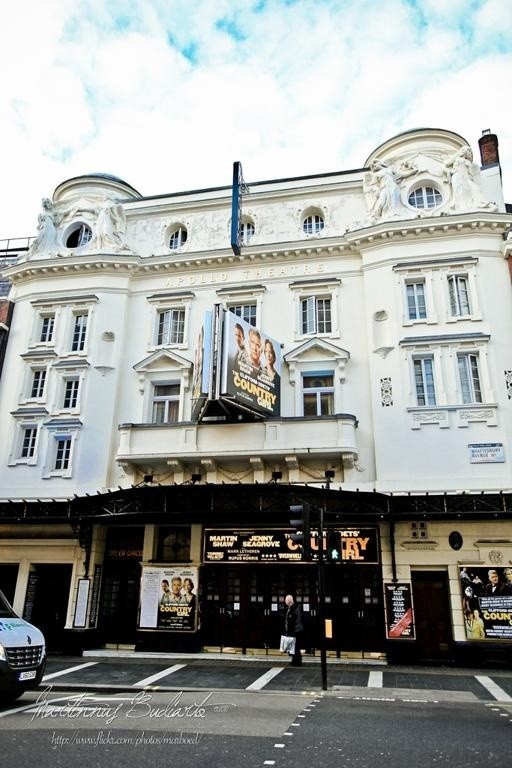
[193,326,203,400]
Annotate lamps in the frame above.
[17,513,21,521]
[134,475,153,488]
[325,470,335,482]
[266,471,281,483]
[191,474,202,485]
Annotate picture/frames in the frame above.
[72,579,91,628]
[382,580,416,641]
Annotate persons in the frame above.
[158,577,194,630]
[233,326,280,409]
[460,568,512,641]
[285,595,304,667]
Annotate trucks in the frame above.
[0,588,47,702]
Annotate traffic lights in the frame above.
[289,501,310,557]
[325,531,342,561]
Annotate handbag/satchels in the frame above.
[280,635,296,654]
